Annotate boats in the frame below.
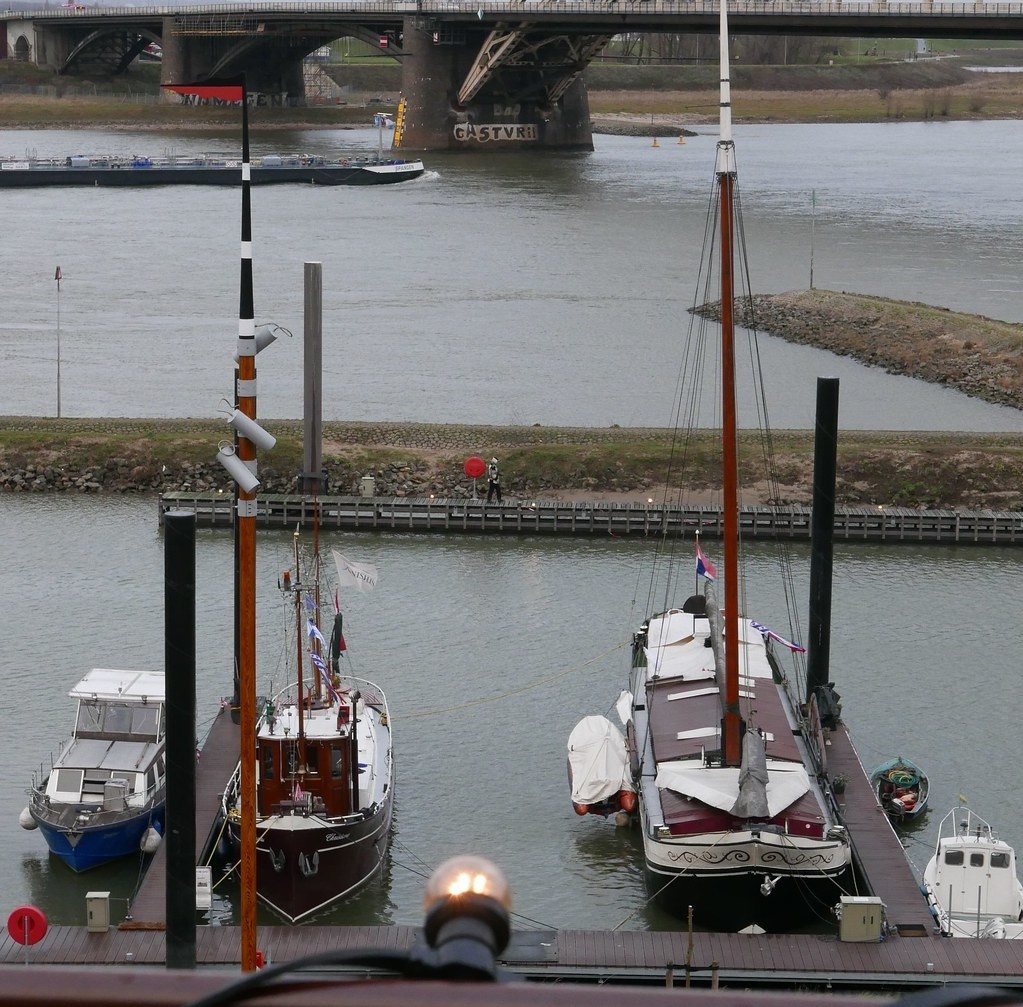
[870,755,930,821]
[23,668,166,876]
[210,260,396,928]
[566,715,636,816]
[0,150,427,186]
[922,803,1023,939]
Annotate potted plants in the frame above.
[832,772,851,794]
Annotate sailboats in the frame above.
[553,0,856,903]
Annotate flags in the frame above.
[305,594,347,705]
[695,543,716,582]
[332,549,378,595]
[295,784,303,802]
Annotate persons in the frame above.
[487,457,504,503]
